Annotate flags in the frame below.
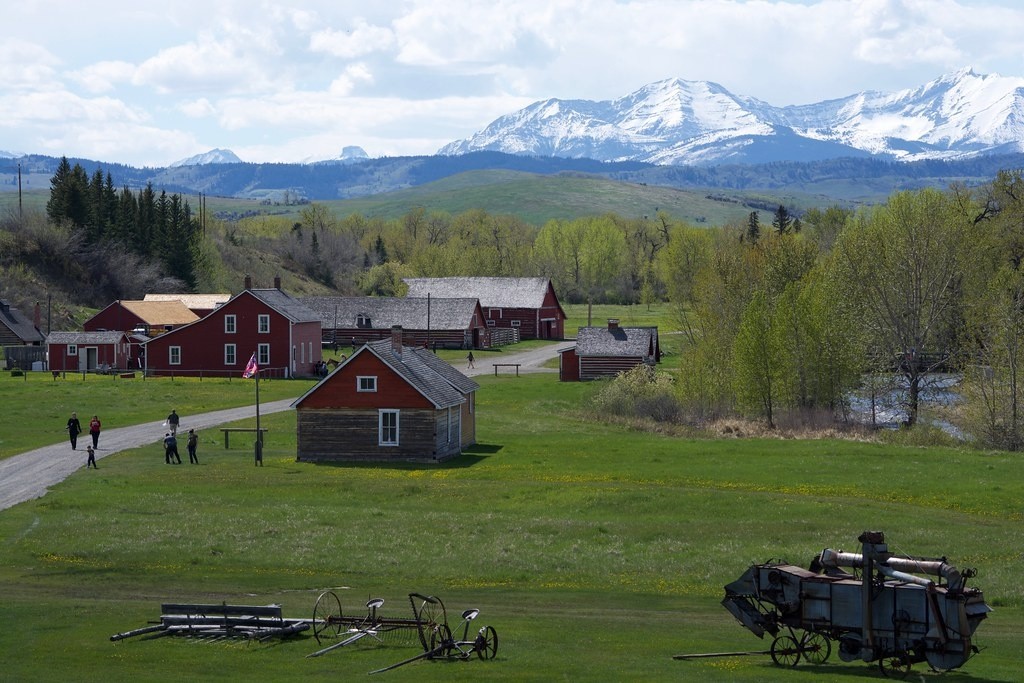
[243,355,258,378]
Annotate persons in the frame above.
[67,412,81,450]
[164,433,182,464]
[90,416,101,449]
[424,338,436,354]
[87,446,96,468]
[466,352,474,369]
[186,429,199,464]
[313,360,328,377]
[167,409,179,437]
[338,354,346,365]
